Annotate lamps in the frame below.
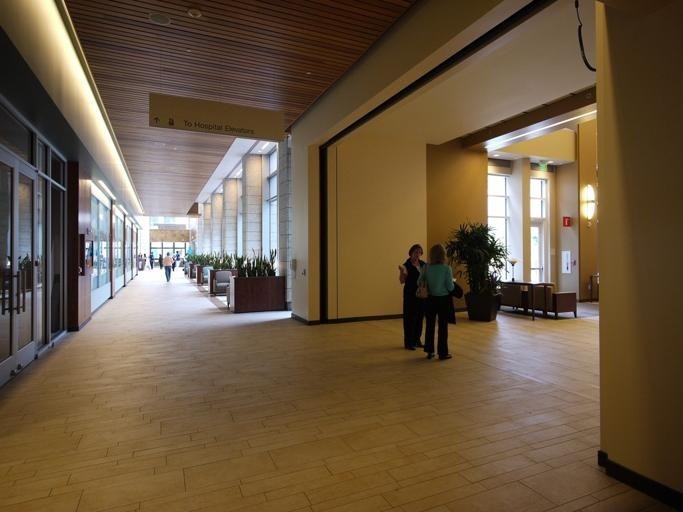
[507,258,517,281]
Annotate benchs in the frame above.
[499,281,577,319]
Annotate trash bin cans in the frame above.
[592,273,599,301]
[138,260,142,270]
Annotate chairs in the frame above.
[185,263,285,314]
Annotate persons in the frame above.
[398,243,426,350]
[416,244,454,361]
[138,251,180,282]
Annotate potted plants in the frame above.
[445,219,512,322]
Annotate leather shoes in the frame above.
[427,354,435,359]
[404,345,416,350]
[416,344,424,348]
[439,354,452,360]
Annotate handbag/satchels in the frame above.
[415,262,429,299]
